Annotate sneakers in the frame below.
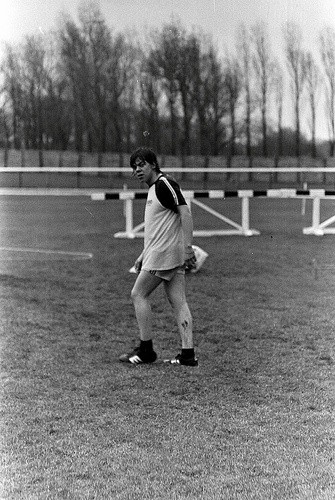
[119,348,158,365]
[163,354,199,366]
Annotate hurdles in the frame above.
[91,188,335,236]
